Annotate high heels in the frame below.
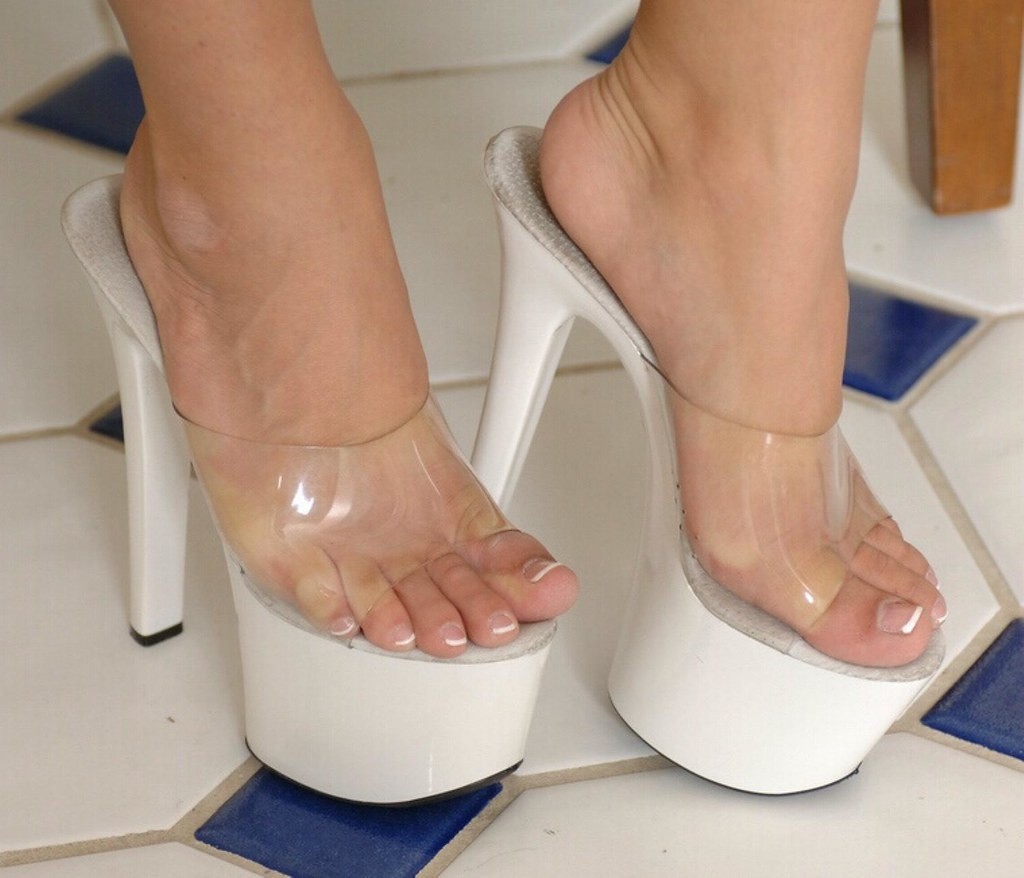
[61,172,560,806]
[470,125,949,796]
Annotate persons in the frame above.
[60,0,954,818]
